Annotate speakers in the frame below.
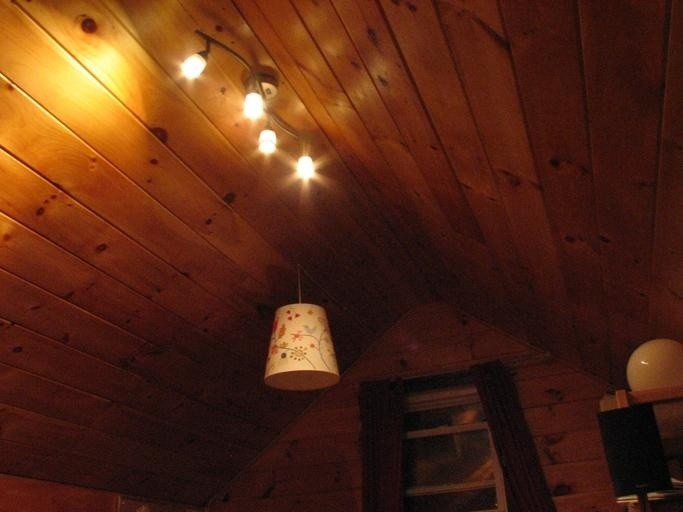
[596,401,673,498]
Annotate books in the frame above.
[614,489,677,500]
[616,497,661,503]
[599,388,635,412]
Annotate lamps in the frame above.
[264,264,341,392]
[181,30,316,182]
